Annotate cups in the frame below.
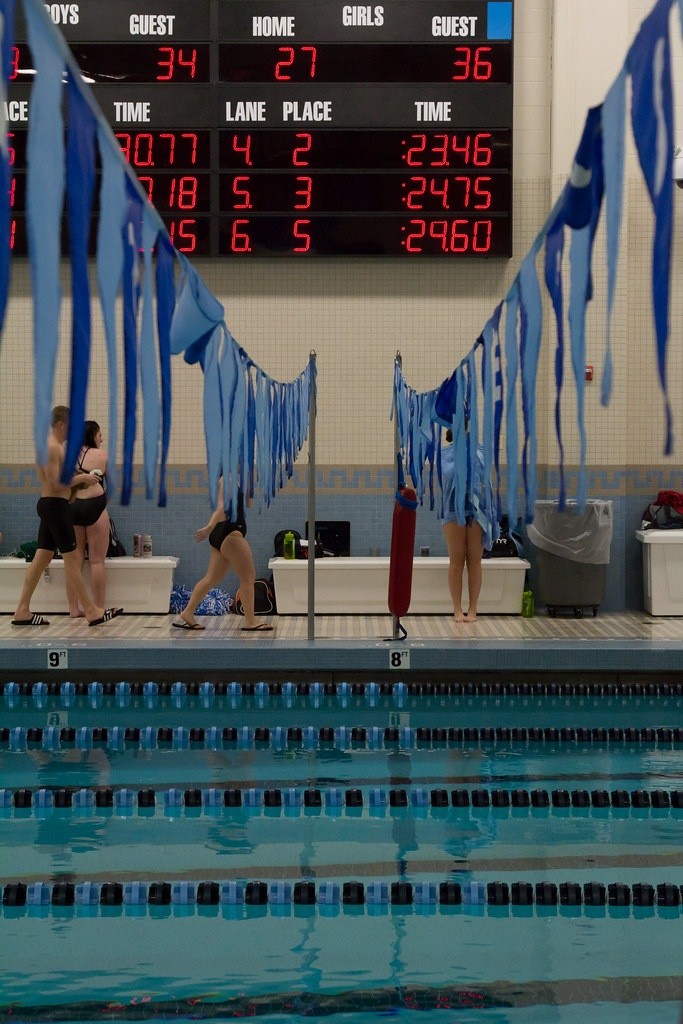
[300,539,317,559]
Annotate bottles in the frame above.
[284,532,295,559]
[133,534,141,557]
[142,535,152,558]
[523,591,533,617]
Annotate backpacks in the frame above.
[83,514,126,559]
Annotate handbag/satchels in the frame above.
[483,514,521,558]
[641,502,683,531]
[236,578,275,615]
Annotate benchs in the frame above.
[268,557,532,615]
[0,555,181,613]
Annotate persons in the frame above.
[65,420,111,618]
[171,458,274,631]
[10,405,124,627]
[437,419,485,622]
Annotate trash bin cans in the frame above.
[533,498,613,618]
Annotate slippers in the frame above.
[171,622,206,630]
[241,623,274,631]
[11,614,50,625]
[89,608,123,627]
